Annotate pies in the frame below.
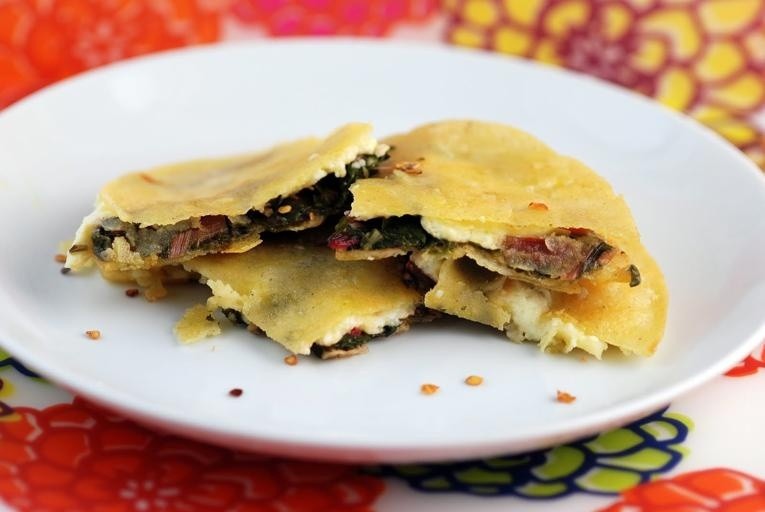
[63,119,665,360]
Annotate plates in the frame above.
[0,37,761,466]
